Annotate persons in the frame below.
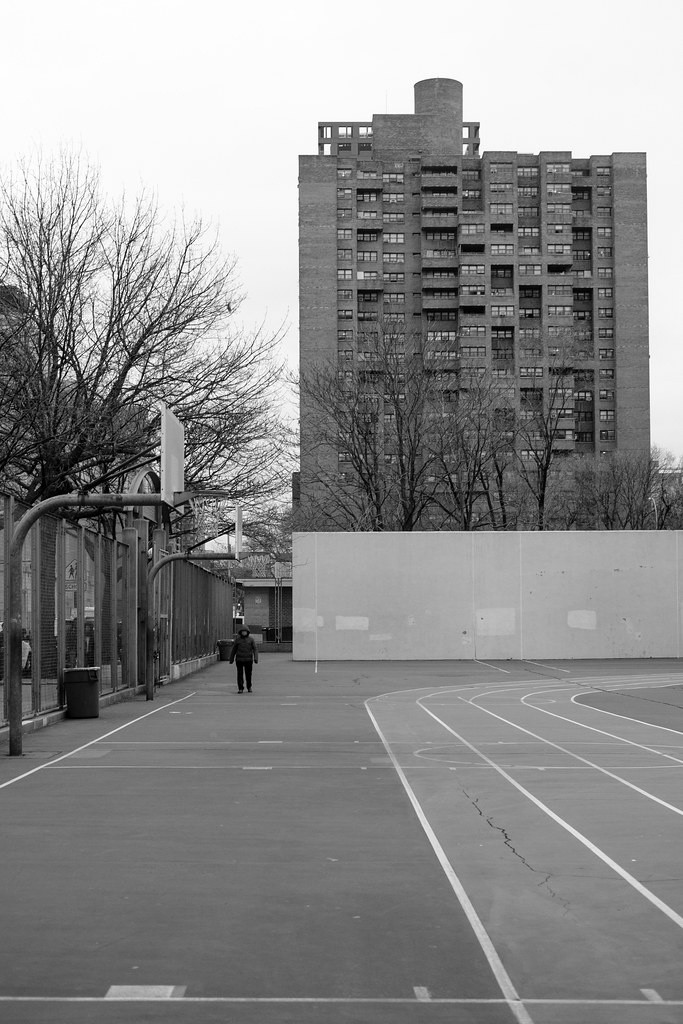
[228,625,259,694]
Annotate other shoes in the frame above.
[248,688,252,692]
[238,689,243,693]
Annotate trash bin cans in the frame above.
[63,667,100,719]
[217,639,235,660]
